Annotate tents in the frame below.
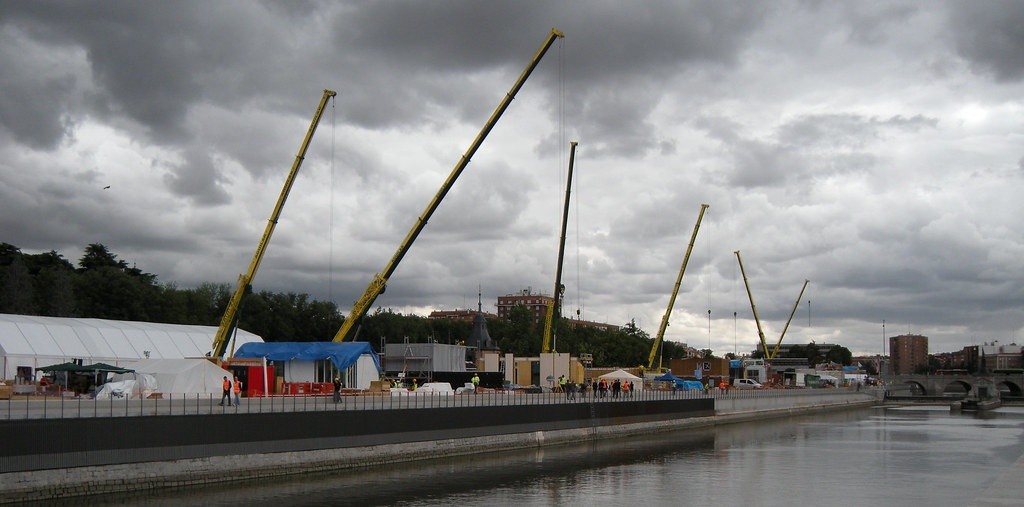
[35,362,135,390]
[112,358,234,399]
[654,371,682,382]
[599,369,642,391]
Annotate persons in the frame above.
[233,376,241,406]
[705,383,708,394]
[560,375,566,392]
[40,378,47,386]
[332,377,342,403]
[565,379,576,400]
[471,373,480,394]
[582,380,587,397]
[672,380,676,395]
[719,379,730,395]
[219,376,232,405]
[857,381,860,391]
[593,379,597,397]
[599,378,634,398]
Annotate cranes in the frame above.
[320,22,571,339]
[207,88,338,363]
[642,203,710,373]
[734,250,811,369]
[540,141,585,353]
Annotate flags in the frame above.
[858,362,862,368]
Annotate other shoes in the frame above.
[228,404,231,406]
[219,403,224,405]
[236,405,240,406]
[233,404,236,406]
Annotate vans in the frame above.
[733,378,764,390]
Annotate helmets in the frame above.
[335,378,338,381]
